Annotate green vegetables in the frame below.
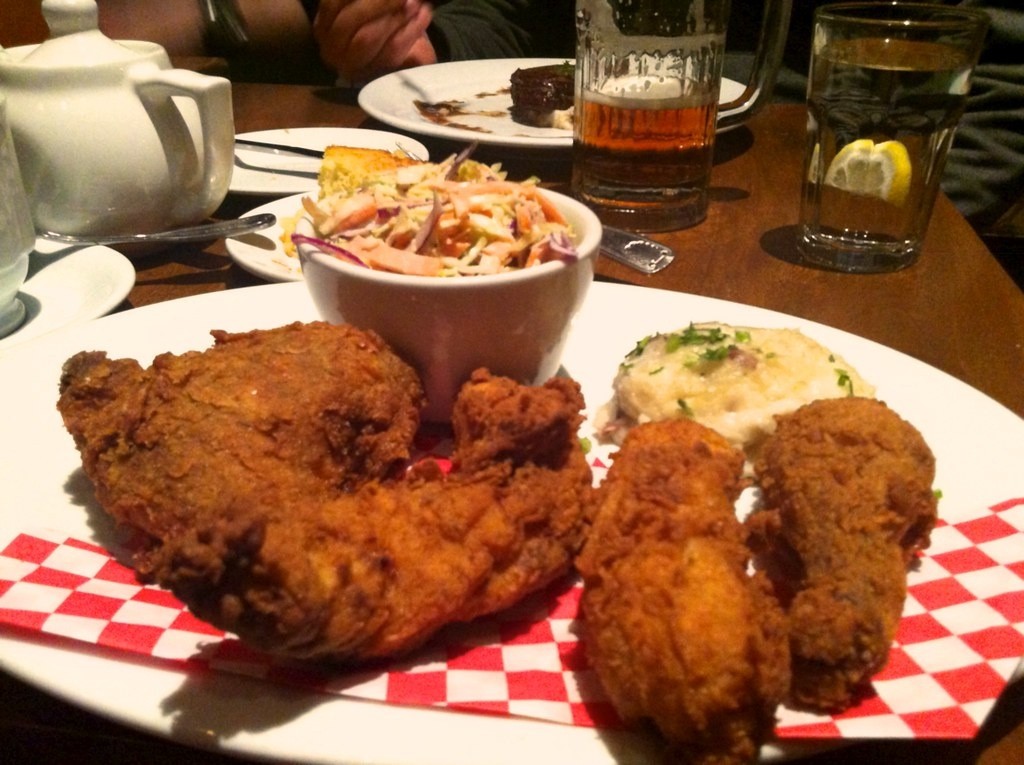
[580,321,853,456]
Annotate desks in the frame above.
[0,58,1024,765]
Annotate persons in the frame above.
[0,0,1024,231]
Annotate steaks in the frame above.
[510,60,577,110]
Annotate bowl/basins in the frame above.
[295,188,601,425]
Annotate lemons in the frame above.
[822,139,911,208]
[808,142,819,184]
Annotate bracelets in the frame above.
[198,0,251,60]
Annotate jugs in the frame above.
[0,0,234,241]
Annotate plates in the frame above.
[227,127,430,197]
[0,238,136,343]
[356,58,756,147]
[0,277,1024,765]
[223,190,321,281]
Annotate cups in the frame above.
[570,0,795,233]
[0,99,31,338]
[797,5,985,276]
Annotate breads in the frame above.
[311,144,427,195]
